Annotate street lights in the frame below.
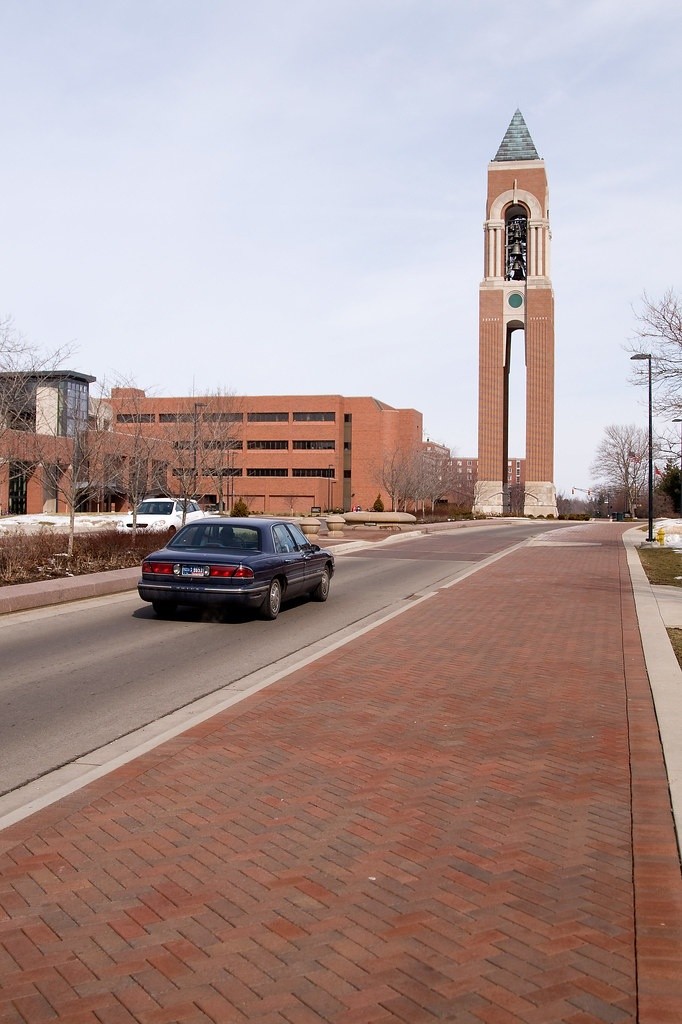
[672,418,682,518]
[331,481,337,513]
[630,354,655,542]
[328,464,334,515]
[193,402,207,502]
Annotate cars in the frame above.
[137,517,336,621]
[624,512,631,518]
[116,498,207,537]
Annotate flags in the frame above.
[655,466,662,475]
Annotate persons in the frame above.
[335,507,344,514]
[221,527,235,547]
[352,505,361,512]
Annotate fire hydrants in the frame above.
[655,526,666,546]
